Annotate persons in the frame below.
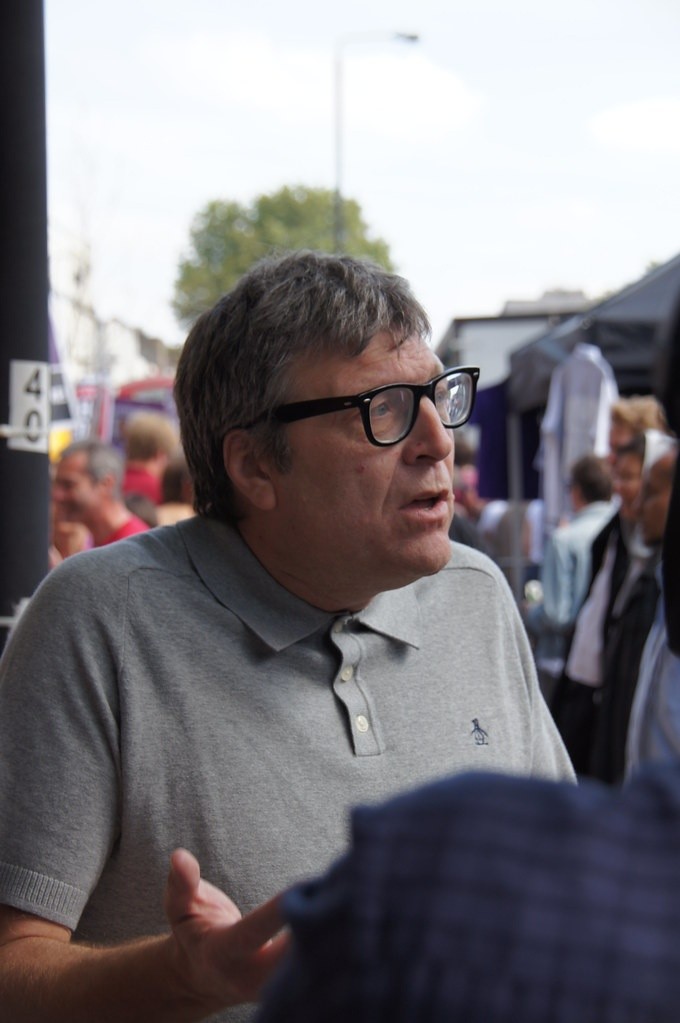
[47,291,680,783]
[246,749,679,1023]
[1,249,583,1023]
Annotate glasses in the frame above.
[216,365,478,452]
